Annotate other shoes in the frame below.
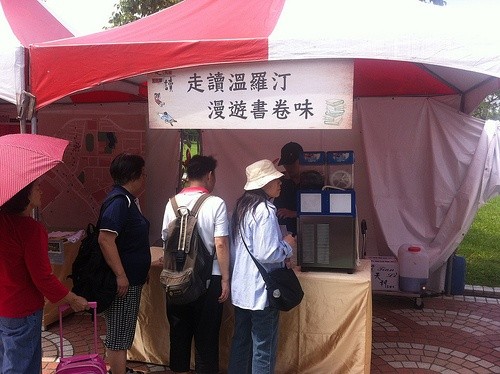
[125,367,144,374]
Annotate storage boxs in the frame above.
[364,255,399,291]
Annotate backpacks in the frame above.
[71,194,130,316]
[159,194,213,301]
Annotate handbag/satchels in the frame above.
[265,266,305,312]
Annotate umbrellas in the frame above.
[0,132,71,206]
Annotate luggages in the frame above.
[59,301,110,374]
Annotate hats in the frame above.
[278,142,303,166]
[243,158,284,190]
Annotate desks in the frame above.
[126,247,372,374]
[42,231,87,331]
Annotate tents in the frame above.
[0,0,500,220]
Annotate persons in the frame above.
[0,180,91,374]
[93,152,152,374]
[160,155,231,374]
[228,157,297,374]
[272,141,325,235]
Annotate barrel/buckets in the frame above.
[451,254,466,294]
[398,243,429,293]
